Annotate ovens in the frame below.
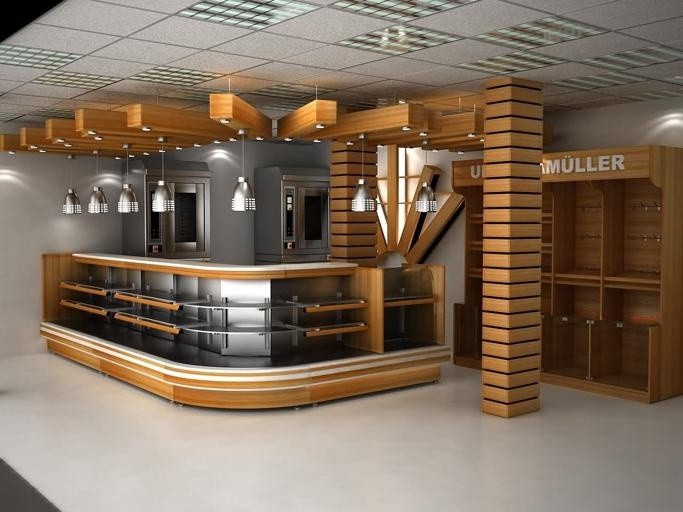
[122,160,213,258]
[255,165,330,263]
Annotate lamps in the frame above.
[416,140,438,212]
[230,129,256,212]
[351,133,377,212]
[62,137,175,215]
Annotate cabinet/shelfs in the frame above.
[451,144,683,404]
[41,253,451,410]
